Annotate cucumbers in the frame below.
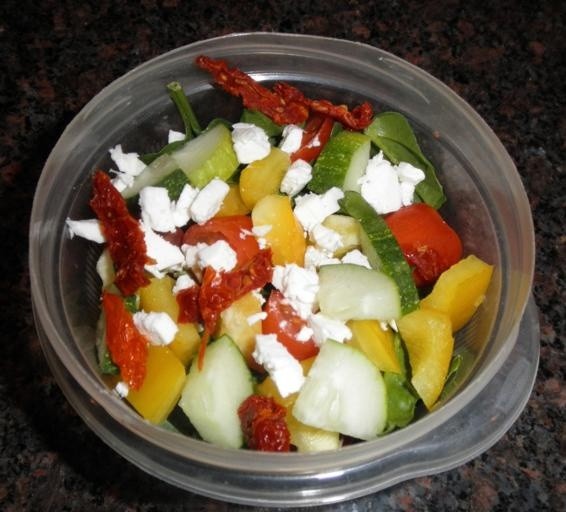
[100,125,421,444]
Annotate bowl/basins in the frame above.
[26,30,544,507]
